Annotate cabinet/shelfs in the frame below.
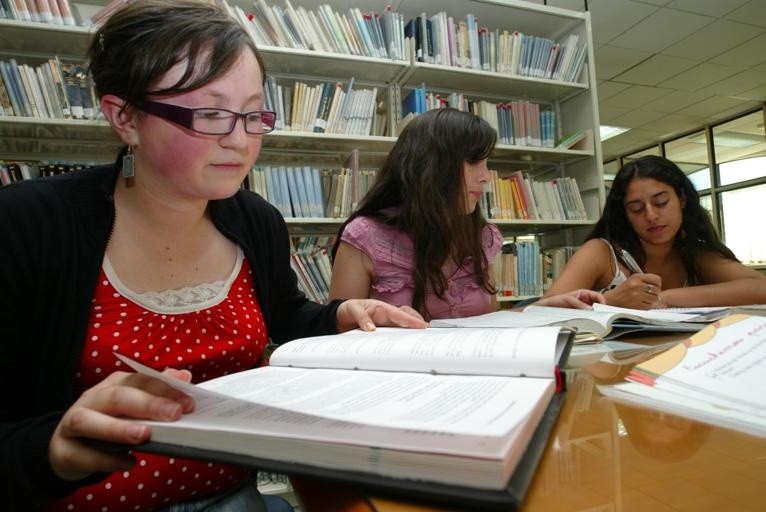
[0,0,607,311]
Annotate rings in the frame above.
[646,284,654,294]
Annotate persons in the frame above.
[537,154,766,310]
[613,404,712,464]
[328,107,504,322]
[0,0,427,511]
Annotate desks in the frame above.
[287,303,766,510]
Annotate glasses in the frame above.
[112,92,278,136]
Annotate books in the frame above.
[494,234,582,297]
[262,72,387,136]
[481,168,589,224]
[0,0,82,27]
[239,161,354,218]
[355,165,380,206]
[428,90,588,152]
[0,158,114,185]
[289,234,338,308]
[592,313,766,441]
[429,299,705,344]
[112,317,580,509]
[221,1,427,60]
[0,54,109,121]
[428,10,587,84]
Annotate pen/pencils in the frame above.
[618,248,644,273]
